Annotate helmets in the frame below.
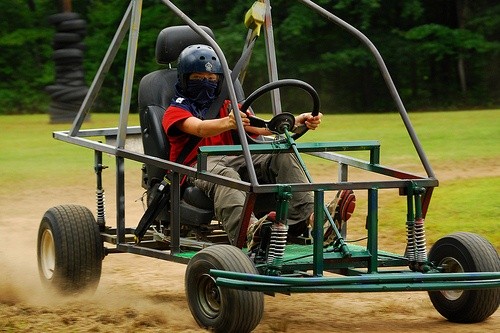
[177,44,223,89]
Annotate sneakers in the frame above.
[247,211,276,252]
[311,190,356,248]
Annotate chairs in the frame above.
[138,26,278,227]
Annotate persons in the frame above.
[161,44,356,266]
